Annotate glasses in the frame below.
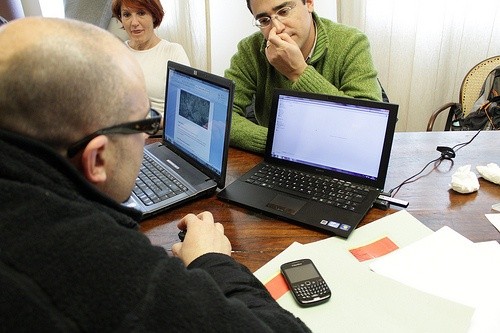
[252,5,296,29]
[67,108,162,159]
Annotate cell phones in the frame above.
[281,259,331,305]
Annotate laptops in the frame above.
[216,87,399,239]
[121,60,235,220]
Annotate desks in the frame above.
[133,131,500,274]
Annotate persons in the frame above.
[224,0,382,154]
[0,17,314,333]
[110,0,189,128]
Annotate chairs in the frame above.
[427,55,500,133]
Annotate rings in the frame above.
[267,45,271,49]
